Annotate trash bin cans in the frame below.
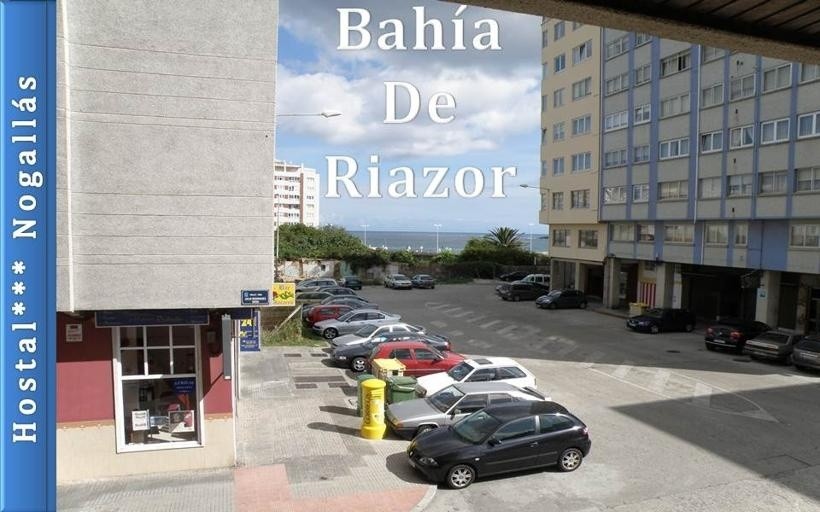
[386,376,418,404]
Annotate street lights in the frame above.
[528,223,535,252]
[360,224,370,246]
[433,224,442,254]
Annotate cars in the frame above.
[743,327,807,367]
[705,319,772,356]
[624,306,696,337]
[790,333,820,375]
[287,273,592,487]
